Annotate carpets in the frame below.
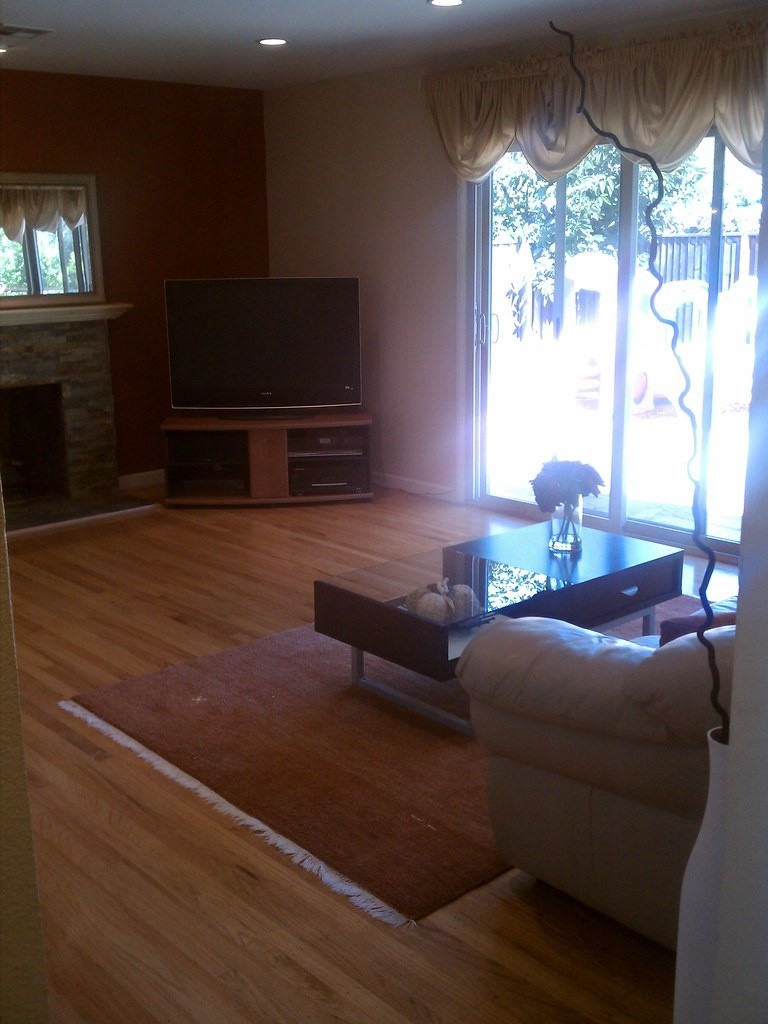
[55,593,717,930]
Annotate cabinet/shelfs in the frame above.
[157,410,376,506]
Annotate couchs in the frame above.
[454,592,738,955]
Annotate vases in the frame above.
[672,725,729,1024]
[546,494,586,553]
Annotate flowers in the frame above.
[526,456,606,514]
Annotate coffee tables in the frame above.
[312,519,683,686]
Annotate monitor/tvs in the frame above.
[164,277,363,419]
